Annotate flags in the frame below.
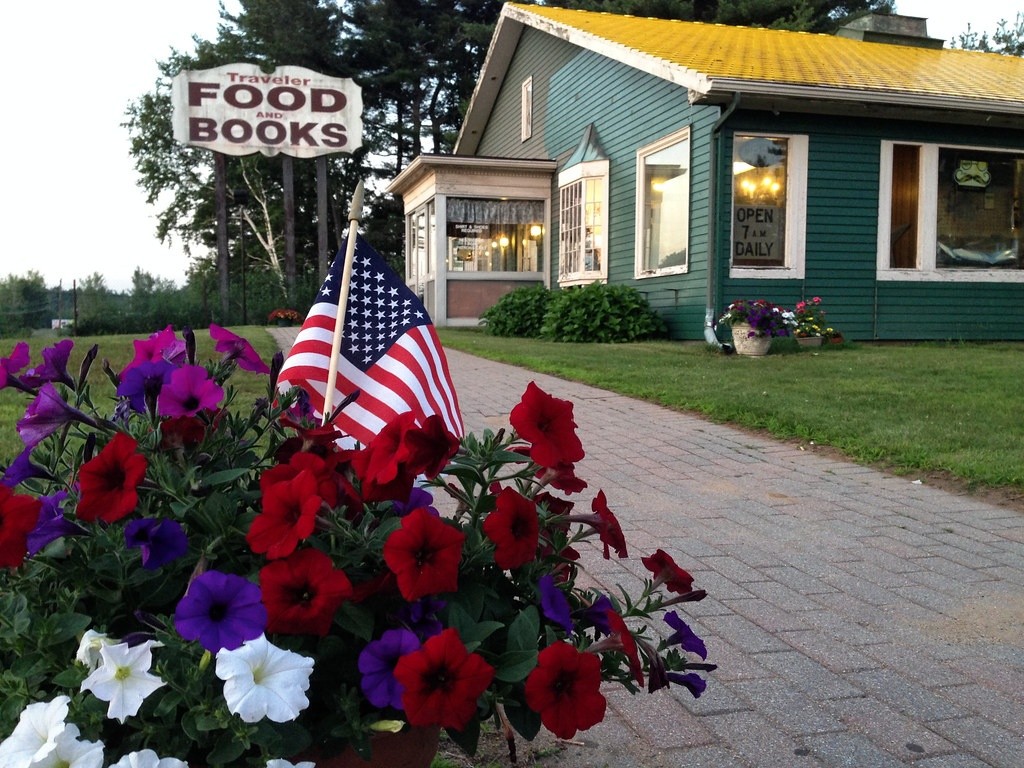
[274,228,465,481]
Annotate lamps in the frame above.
[652,177,669,193]
[740,175,782,197]
[485,251,489,256]
[491,241,499,250]
[499,237,510,248]
[529,224,542,237]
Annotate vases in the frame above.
[732,327,773,356]
[797,337,824,350]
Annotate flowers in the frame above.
[794,296,836,338]
[0,321,720,768]
[719,299,798,338]
